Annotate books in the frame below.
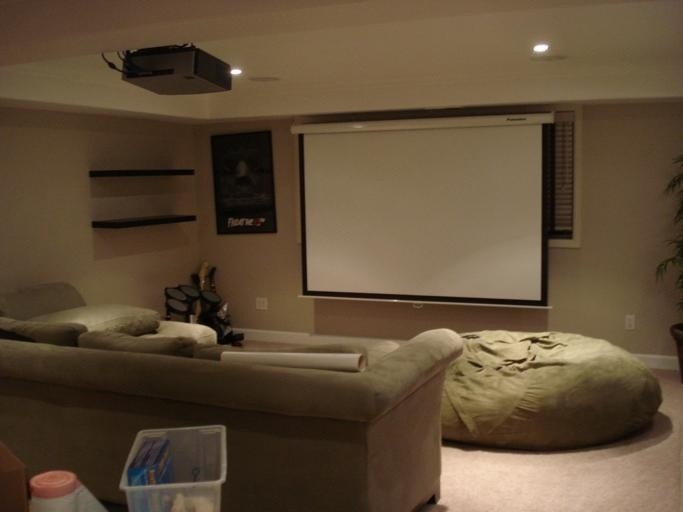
[126,437,176,512]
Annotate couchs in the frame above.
[1,282,463,511]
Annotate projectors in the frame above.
[122,45,232,97]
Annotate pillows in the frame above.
[1,318,90,345]
[193,336,367,374]
[76,326,199,356]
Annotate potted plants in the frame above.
[652,153,683,384]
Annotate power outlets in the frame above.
[624,313,637,331]
